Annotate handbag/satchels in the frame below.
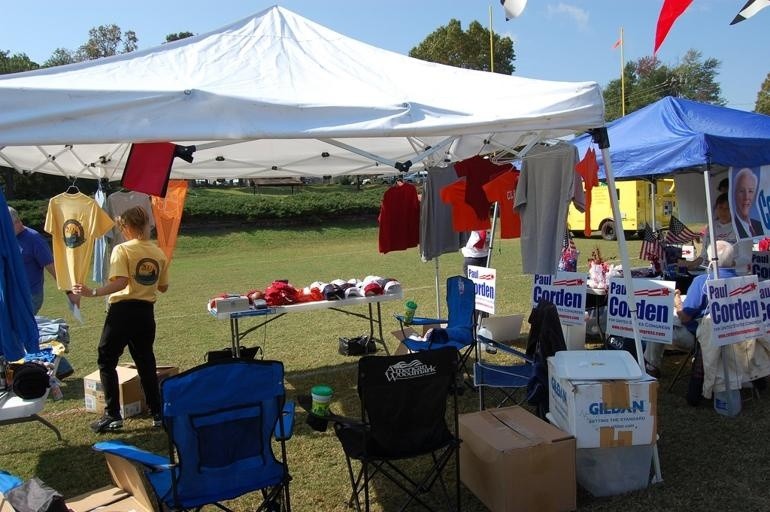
[429,327,448,344]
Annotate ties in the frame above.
[747,222,756,237]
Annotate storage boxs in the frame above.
[545,411,660,496]
[61,451,160,512]
[452,405,580,511]
[546,355,661,448]
[82,360,181,421]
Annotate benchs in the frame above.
[252,178,303,196]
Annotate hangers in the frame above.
[64,172,131,195]
[389,128,597,189]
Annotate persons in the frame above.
[644,240,741,377]
[460,229,491,325]
[733,167,765,239]
[7,205,82,316]
[71,205,171,434]
[699,193,738,267]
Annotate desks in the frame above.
[204,289,403,360]
[584,292,609,347]
[1,330,62,445]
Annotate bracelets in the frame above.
[65,291,74,296]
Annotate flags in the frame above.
[653,0,692,52]
[730,0,770,26]
[500,0,533,21]
[638,215,701,269]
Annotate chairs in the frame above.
[299,346,465,512]
[393,276,481,399]
[472,300,564,424]
[89,359,296,512]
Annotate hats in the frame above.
[310,276,403,301]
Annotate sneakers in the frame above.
[153,415,161,427]
[90,415,123,433]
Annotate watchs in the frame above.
[92,287,97,296]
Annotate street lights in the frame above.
[488,0,529,74]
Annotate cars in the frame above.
[360,172,428,186]
[196,178,241,187]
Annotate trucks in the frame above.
[568,179,676,241]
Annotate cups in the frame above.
[310,385,334,415]
[403,302,417,324]
